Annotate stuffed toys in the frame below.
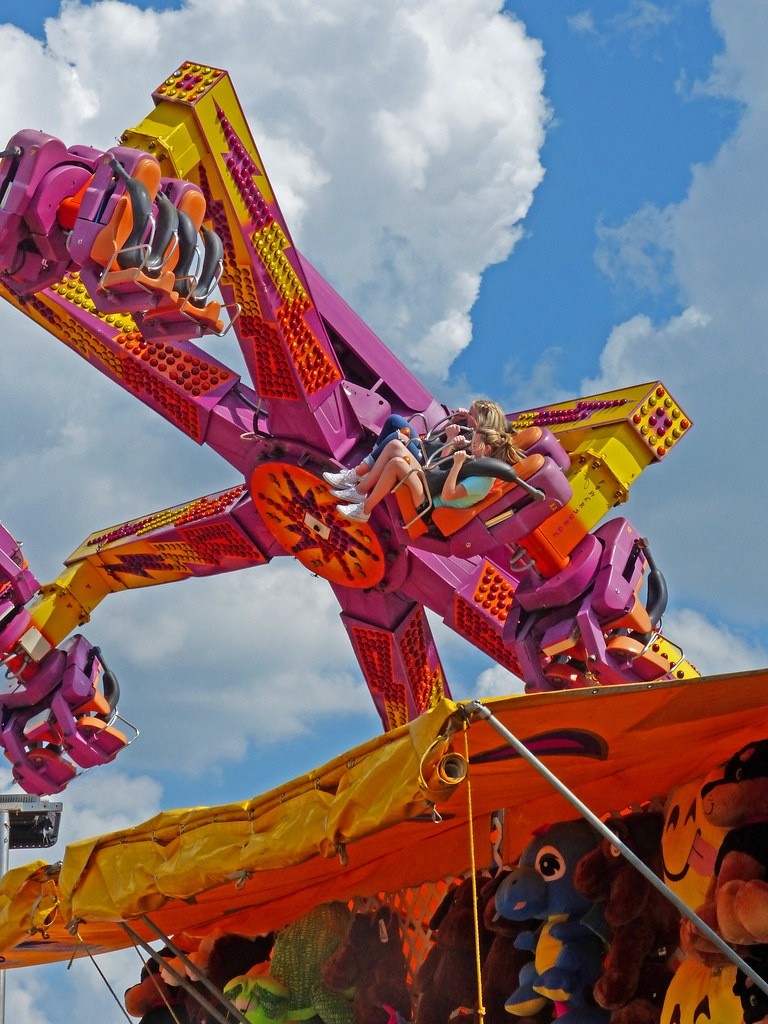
[124,740,768,1024]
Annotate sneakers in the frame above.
[323,471,360,489]
[339,469,369,482]
[336,502,371,523]
[329,487,368,503]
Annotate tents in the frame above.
[1,666,768,1024]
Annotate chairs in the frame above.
[383,412,572,558]
[0,525,140,797]
[603,631,685,678]
[0,129,243,341]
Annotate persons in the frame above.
[322,400,522,522]
